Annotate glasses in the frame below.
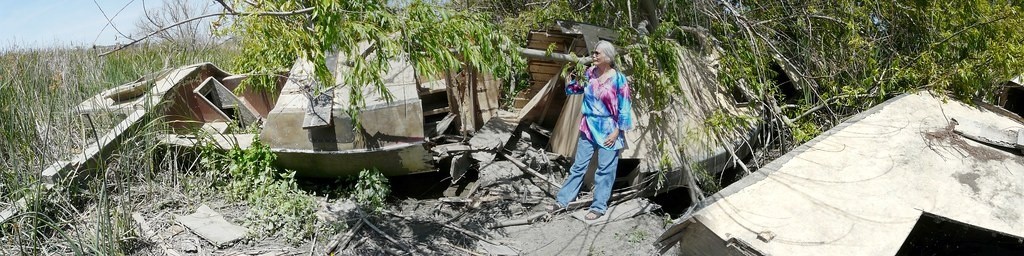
[591,51,601,55]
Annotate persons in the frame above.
[545,40,632,221]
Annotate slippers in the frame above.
[545,203,563,212]
[584,210,602,220]
